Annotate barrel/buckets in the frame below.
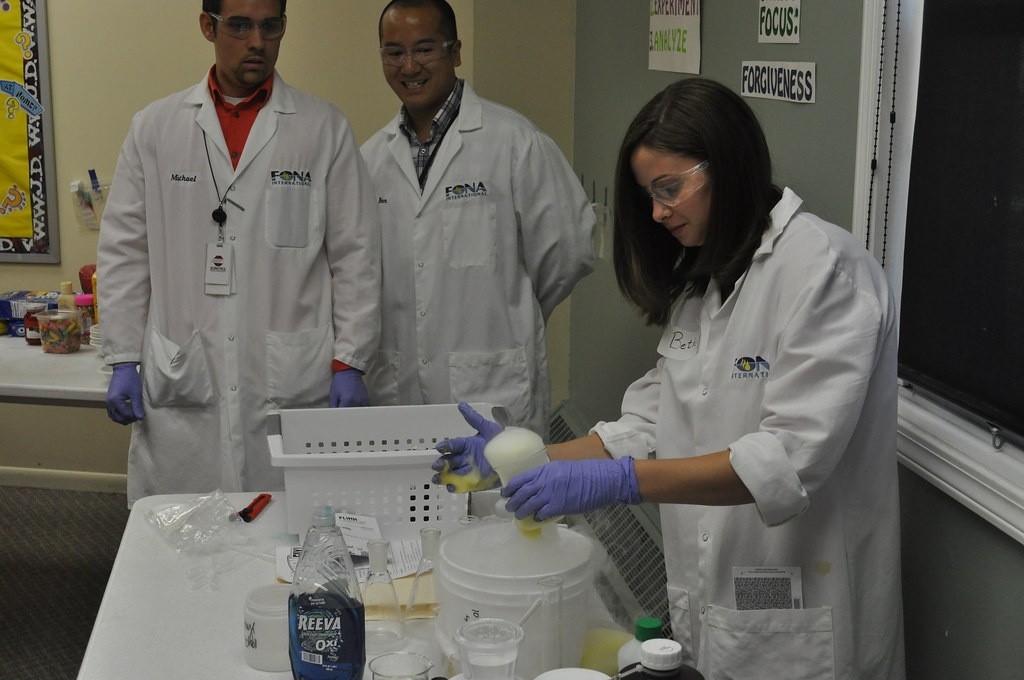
[440,521,597,680]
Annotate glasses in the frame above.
[644,161,710,206]
[378,40,457,65]
[208,12,287,41]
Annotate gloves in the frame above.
[329,369,369,407]
[503,455,637,523]
[105,363,146,425]
[430,399,505,496]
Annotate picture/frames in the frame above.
[0,0,63,267]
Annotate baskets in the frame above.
[266,401,493,538]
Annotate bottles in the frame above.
[617,617,663,675]
[363,538,412,652]
[404,524,444,643]
[288,483,366,680]
[618,639,706,680]
[58,282,77,312]
[24,304,47,346]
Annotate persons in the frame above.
[430,74,908,679]
[351,0,595,451]
[91,0,386,509]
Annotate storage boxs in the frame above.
[35,308,85,353]
[0,289,62,319]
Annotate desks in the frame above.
[78,488,617,680]
[0,333,133,476]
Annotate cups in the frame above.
[243,584,292,671]
[369,652,434,680]
